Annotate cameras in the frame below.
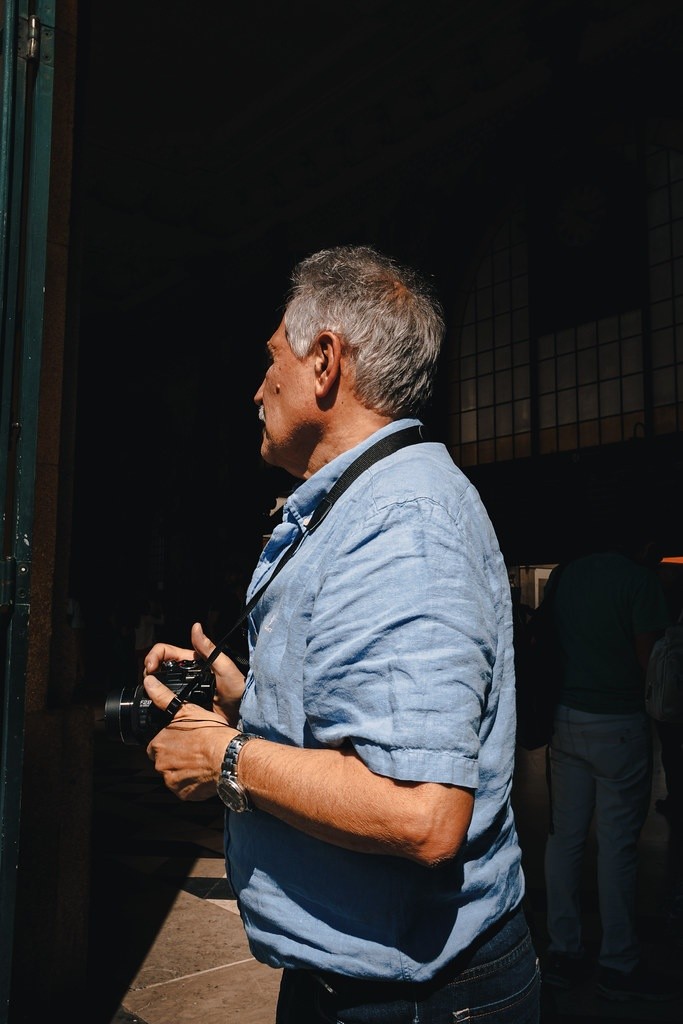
[103,656,216,753]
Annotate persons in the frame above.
[204,559,250,679]
[142,237,545,1023]
[132,600,165,684]
[540,514,683,1001]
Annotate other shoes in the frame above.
[654,795,683,809]
[544,947,606,986]
[605,957,682,1001]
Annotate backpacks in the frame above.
[515,558,572,750]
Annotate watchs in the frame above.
[216,731,266,814]
